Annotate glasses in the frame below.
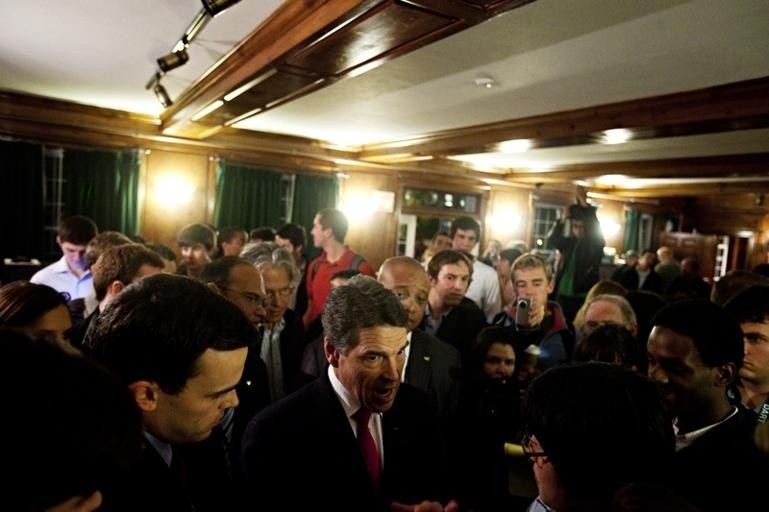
[218,283,272,308]
[264,283,295,299]
[519,435,547,464]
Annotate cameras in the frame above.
[511,294,533,329]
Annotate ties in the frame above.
[350,408,384,488]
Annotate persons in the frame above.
[711,268,768,304]
[496,248,521,312]
[29,214,97,300]
[237,242,306,415]
[421,232,451,270]
[723,284,769,420]
[548,208,605,322]
[517,362,673,512]
[303,208,374,332]
[235,278,450,512]
[66,243,166,348]
[377,255,456,407]
[461,337,520,421]
[583,295,637,335]
[671,258,711,297]
[653,247,681,280]
[419,250,474,352]
[217,226,249,258]
[46,273,261,506]
[645,300,769,512]
[610,250,638,281]
[426,217,502,324]
[572,322,637,372]
[249,226,275,243]
[300,269,361,380]
[574,280,626,330]
[0,280,81,355]
[621,249,664,293]
[197,256,265,331]
[72,232,134,322]
[173,222,216,279]
[273,223,313,315]
[483,240,501,265]
[491,253,573,400]
